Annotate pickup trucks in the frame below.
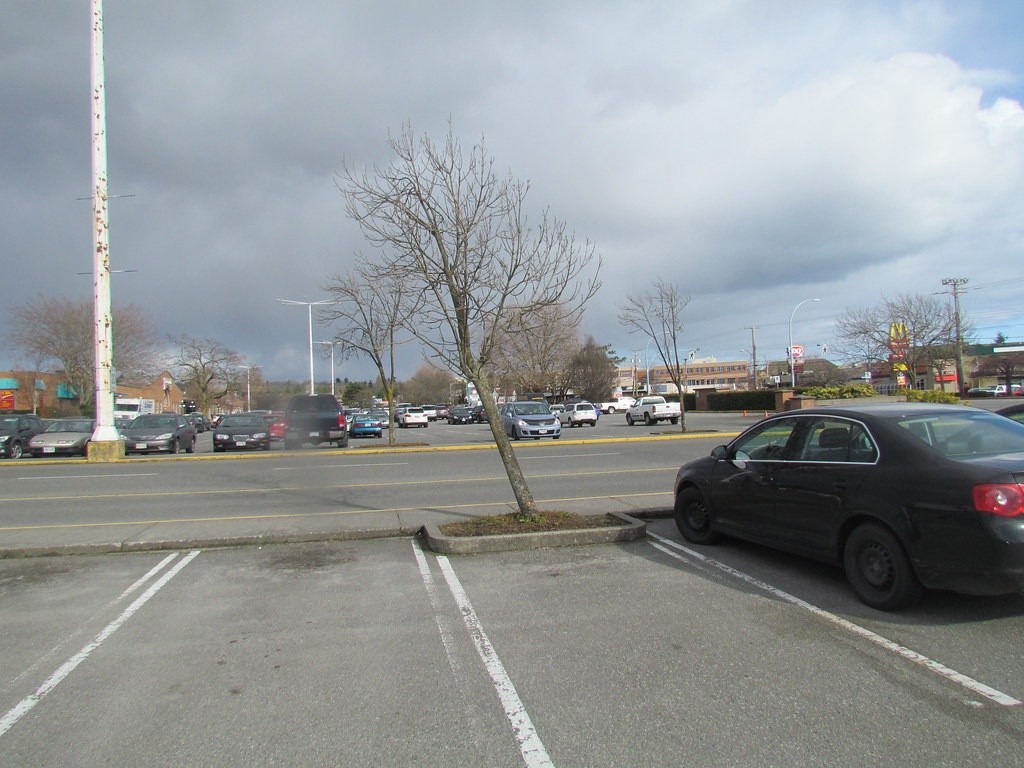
[593,397,639,414]
[626,396,681,426]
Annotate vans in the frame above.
[516,394,549,407]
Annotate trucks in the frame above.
[114,399,155,421]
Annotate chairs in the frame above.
[811,428,856,462]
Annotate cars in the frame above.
[967,385,1024,397]
[674,402,1024,611]
[501,402,560,441]
[29,418,96,456]
[549,404,566,416]
[212,410,284,453]
[344,407,388,440]
[119,414,197,455]
[181,412,225,434]
[0,413,45,459]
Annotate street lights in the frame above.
[740,350,757,390]
[789,299,820,387]
[645,332,669,395]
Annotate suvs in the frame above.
[555,402,597,427]
[395,403,487,429]
[283,394,347,449]
